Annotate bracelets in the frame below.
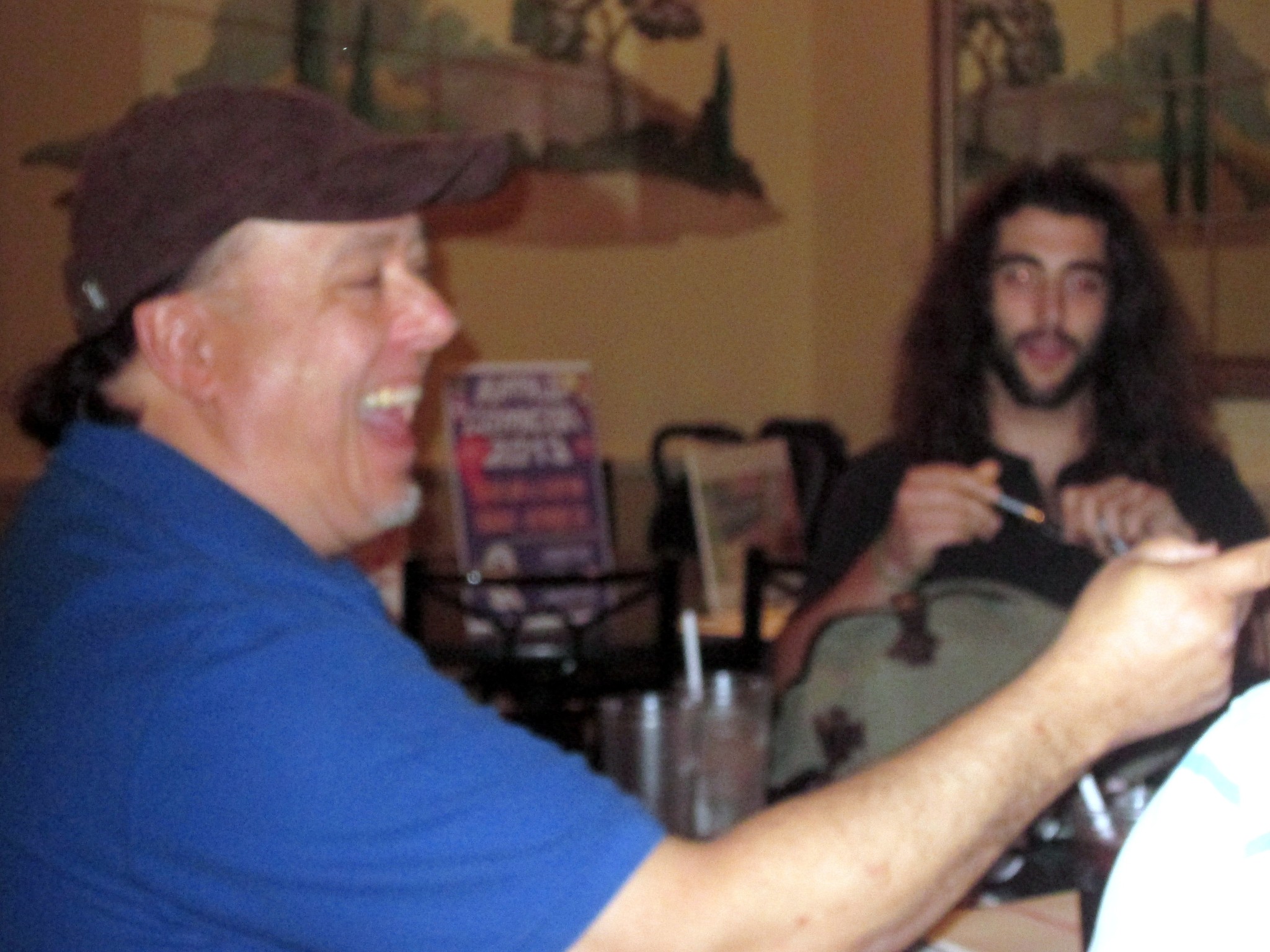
[865,539,918,593]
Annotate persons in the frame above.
[771,157,1270,696]
[0,81,1270,952]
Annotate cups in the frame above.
[1069,796,1151,952]
[601,693,673,833]
[673,669,773,843]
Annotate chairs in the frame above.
[411,416,849,755]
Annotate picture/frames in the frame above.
[932,0,1269,398]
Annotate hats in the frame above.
[64,81,513,334]
